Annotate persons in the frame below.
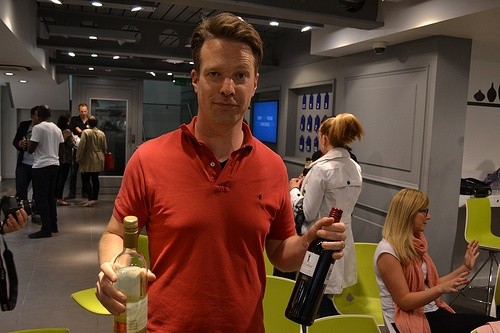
[96,12,348,333]
[14,103,108,238]
[288,112,363,316]
[373,188,496,333]
[0,209,29,235]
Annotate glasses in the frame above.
[419,208,429,216]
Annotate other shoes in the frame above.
[31,213,41,224]
[51,226,59,233]
[28,229,52,238]
[56,199,71,206]
[81,200,97,206]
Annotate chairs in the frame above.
[446,197,500,316]
[262,238,390,333]
[72,233,150,333]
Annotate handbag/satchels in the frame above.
[104,152,115,170]
[273,210,306,280]
[460,178,492,197]
[0,234,18,312]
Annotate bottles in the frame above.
[473,82,500,103]
[285,207,344,326]
[303,158,313,188]
[113,215,149,333]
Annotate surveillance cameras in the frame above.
[373,40,386,54]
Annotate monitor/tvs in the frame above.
[252,100,278,144]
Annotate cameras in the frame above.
[0,195,37,220]
